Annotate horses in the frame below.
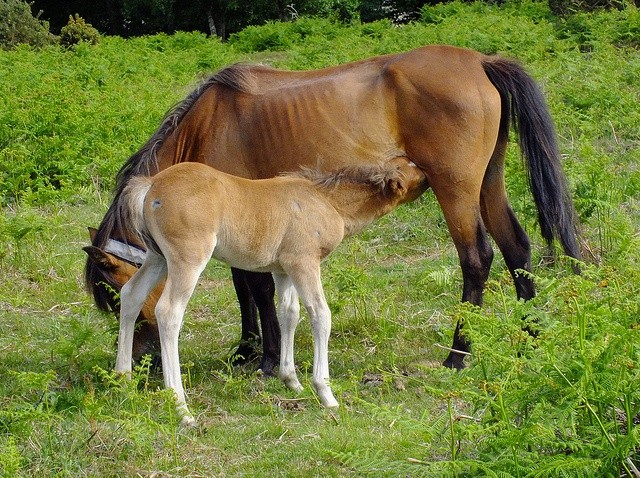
[80,44,588,378]
[113,154,430,429]
